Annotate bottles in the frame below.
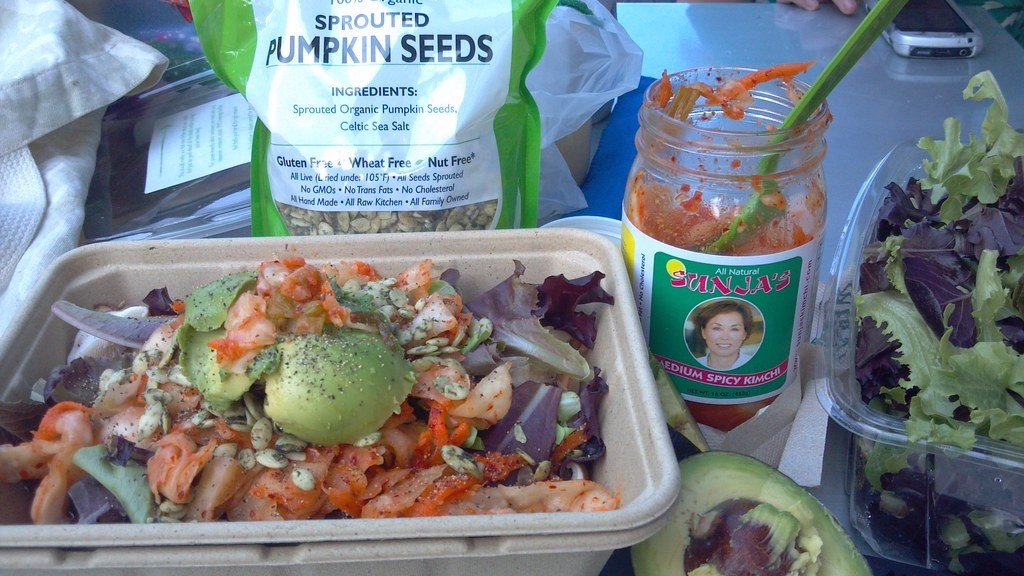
[617,64,834,441]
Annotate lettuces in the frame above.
[855,65,1024,576]
[0,257,618,521]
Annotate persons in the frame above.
[696,300,752,371]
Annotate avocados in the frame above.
[631,450,875,576]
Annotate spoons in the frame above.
[691,0,907,255]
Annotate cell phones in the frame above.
[866,0,983,58]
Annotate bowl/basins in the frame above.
[810,136,1024,576]
[0,227,682,576]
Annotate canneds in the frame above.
[619,65,831,429]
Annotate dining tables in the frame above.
[618,0,1024,342]
